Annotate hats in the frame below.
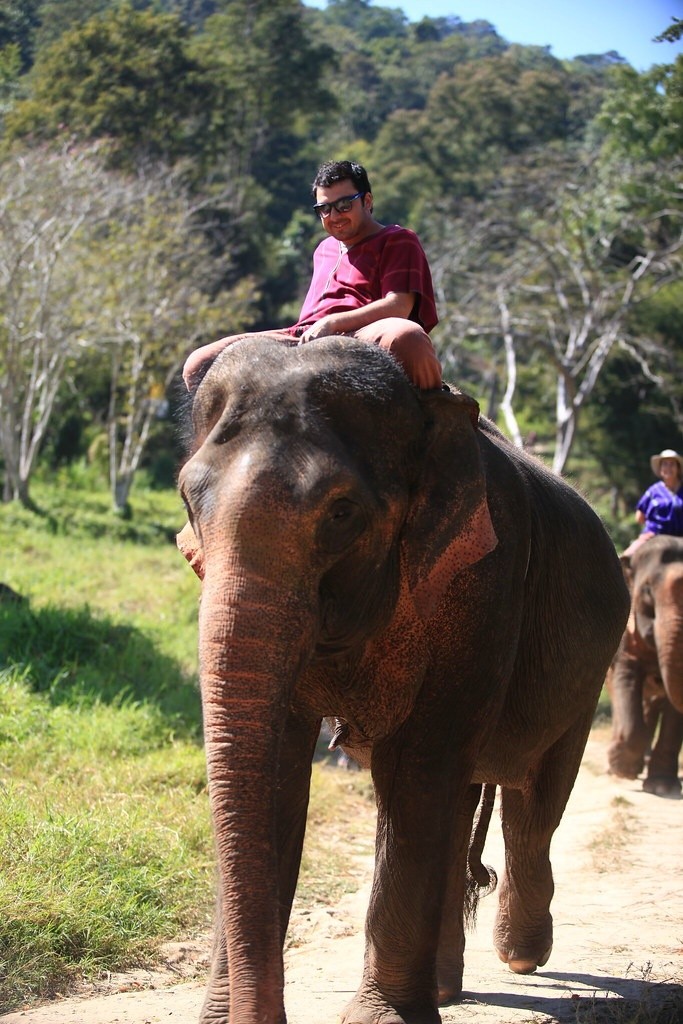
[650,449,683,477]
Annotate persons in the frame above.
[181,160,443,395]
[622,449,683,558]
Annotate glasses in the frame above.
[312,190,367,218]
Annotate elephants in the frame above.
[607,534,683,801]
[174,331,632,1024]
